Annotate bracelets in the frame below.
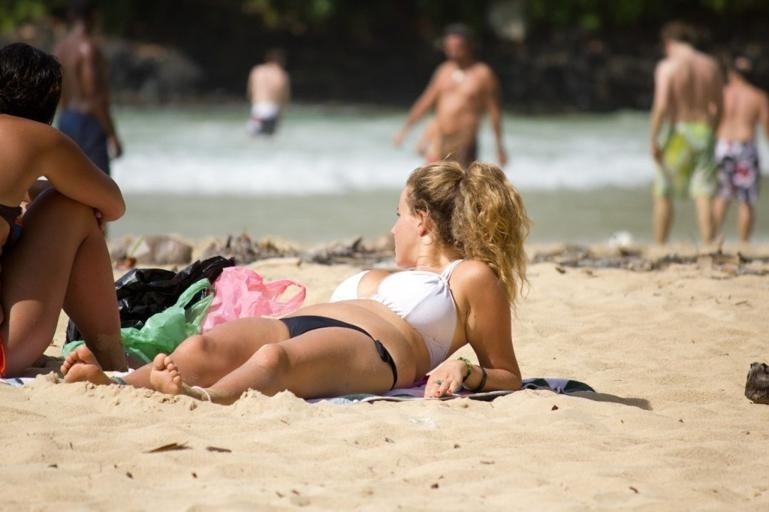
[467,364,488,394]
[456,357,471,384]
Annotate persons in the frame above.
[0,40,137,381]
[61,160,522,404]
[248,43,293,142]
[46,3,121,176]
[394,18,508,171]
[702,55,768,246]
[647,22,723,247]
